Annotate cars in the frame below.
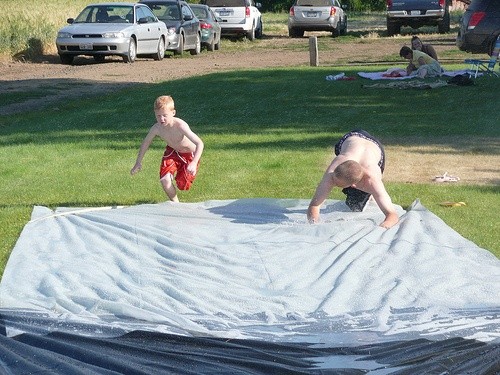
[55,2,170,63]
[163,4,223,52]
[287,0,349,38]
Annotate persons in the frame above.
[306,129,399,230]
[406,36,438,75]
[130,95,204,203]
[400,46,441,78]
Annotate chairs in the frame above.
[96,10,108,22]
[126,13,134,23]
[464,32,500,79]
[163,4,180,20]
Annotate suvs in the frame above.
[455,0,500,63]
[386,0,452,34]
[125,0,203,56]
[206,0,264,41]
